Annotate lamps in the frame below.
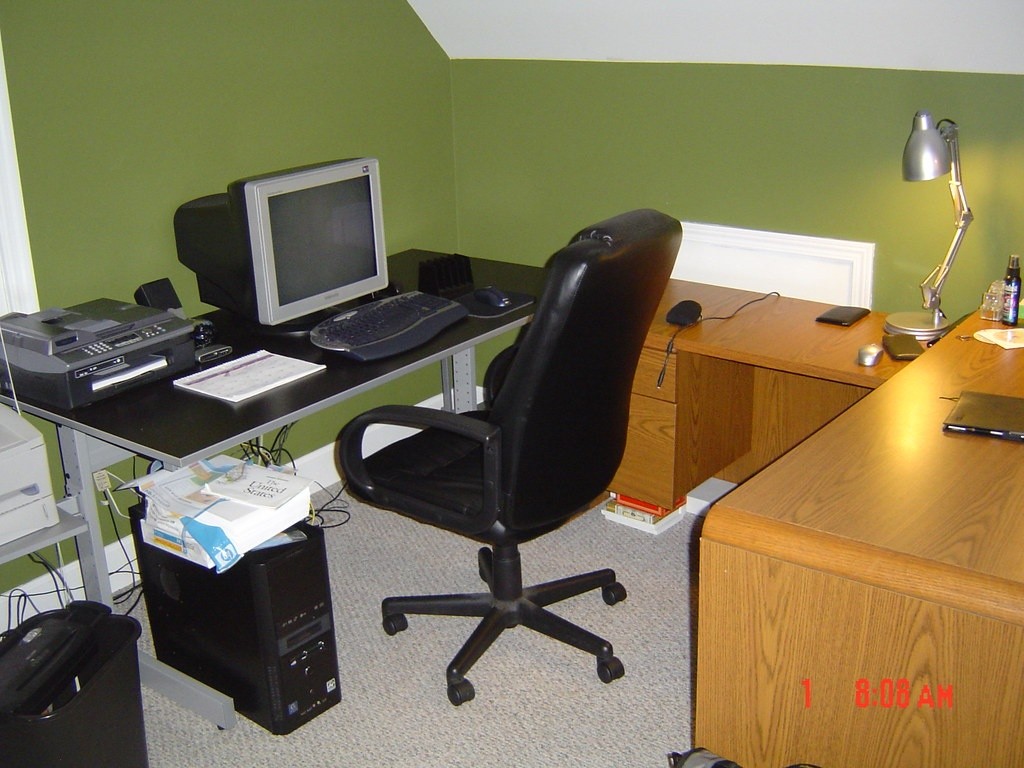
[880,107,977,341]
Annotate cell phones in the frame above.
[814,307,871,327]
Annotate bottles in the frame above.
[1001,256,1021,326]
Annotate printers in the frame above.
[0,405,60,546]
[0,298,197,410]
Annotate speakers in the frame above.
[134,277,187,321]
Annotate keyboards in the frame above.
[310,290,470,362]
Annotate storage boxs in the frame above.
[139,455,311,569]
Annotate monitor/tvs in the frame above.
[174,157,389,337]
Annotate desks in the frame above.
[596,278,935,510]
[689,309,1024,768]
[0,246,551,729]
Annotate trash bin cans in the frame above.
[1,598,152,768]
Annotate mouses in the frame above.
[857,344,884,366]
[473,285,512,308]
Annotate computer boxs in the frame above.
[130,500,343,735]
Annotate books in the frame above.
[942,391,1024,443]
[600,491,686,536]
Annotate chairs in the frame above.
[335,210,681,707]
